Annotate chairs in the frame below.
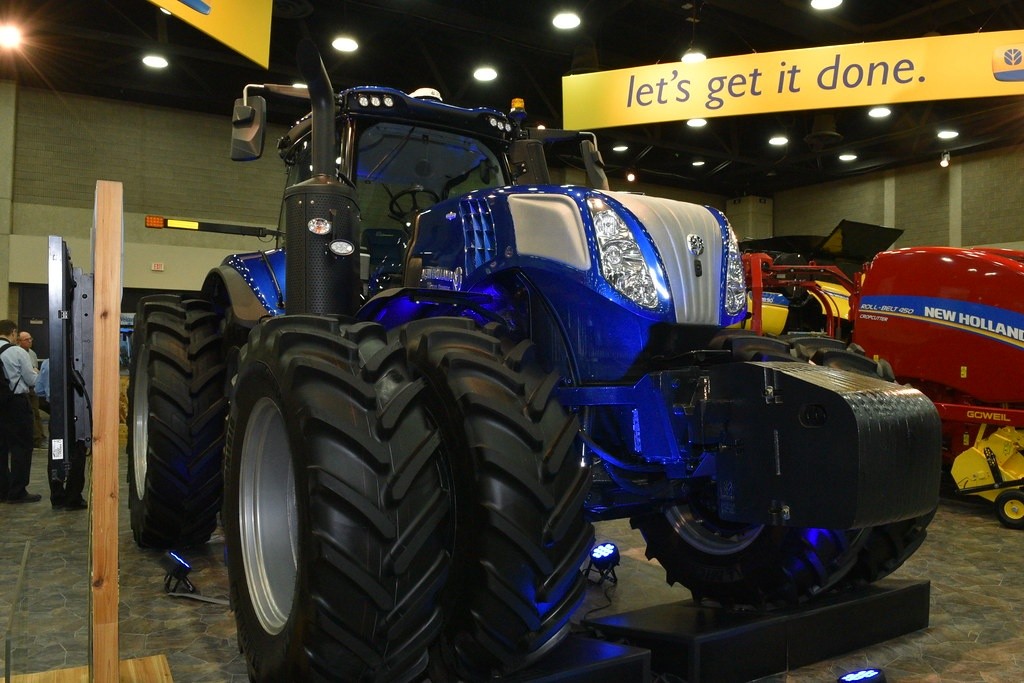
[361,229,408,278]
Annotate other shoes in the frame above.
[7,494,42,504]
[32,438,48,449]
[52,500,88,511]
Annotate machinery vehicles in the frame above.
[124,48,943,683]
[741,217,1024,498]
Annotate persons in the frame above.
[34,356,90,510]
[0,318,42,504]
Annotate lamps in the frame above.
[585,543,621,587]
[158,551,196,595]
[681,16,707,62]
[803,113,843,146]
[563,35,600,76]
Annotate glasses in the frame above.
[20,338,33,341]
